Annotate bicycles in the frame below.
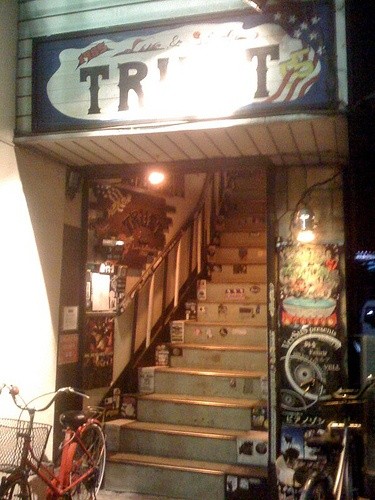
[0,382,107,500]
[293,372,375,500]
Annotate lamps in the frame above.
[295,195,317,244]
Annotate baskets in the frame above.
[1,418,52,477]
